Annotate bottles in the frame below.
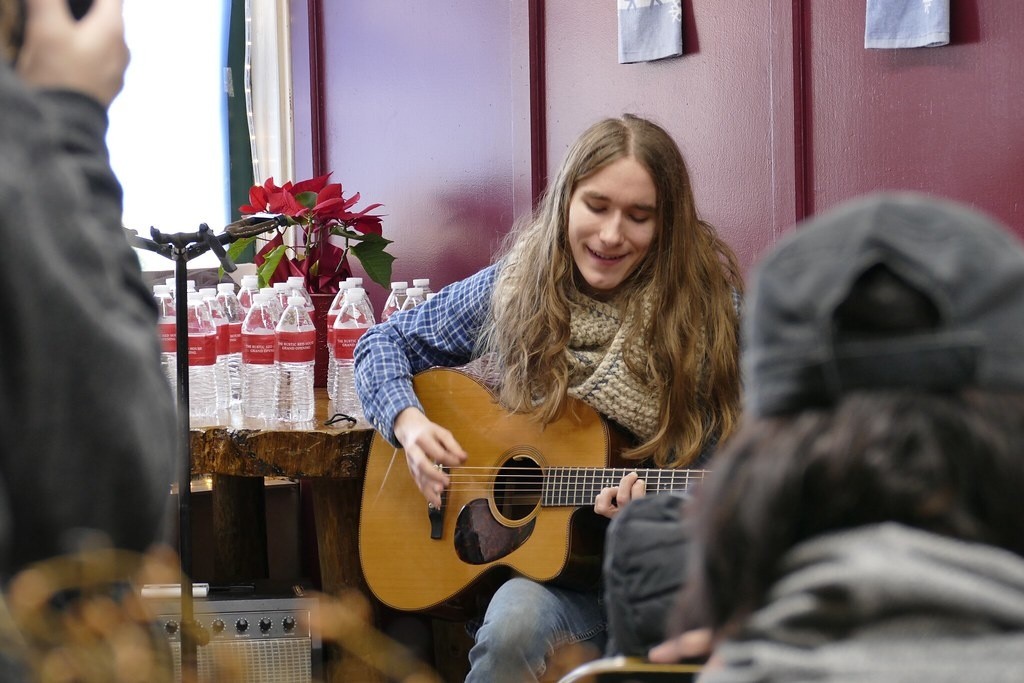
[412,279,432,300]
[334,288,376,425]
[401,288,425,311]
[151,284,177,413]
[236,274,259,316]
[381,281,408,323]
[165,278,176,305]
[187,280,231,427]
[242,294,275,429]
[272,276,315,326]
[426,292,437,301]
[275,297,316,423]
[345,277,375,314]
[326,281,356,401]
[215,282,246,405]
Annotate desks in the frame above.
[189,388,397,683]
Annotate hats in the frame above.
[741,192,1024,422]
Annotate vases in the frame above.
[311,296,335,388]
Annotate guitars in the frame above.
[358,368,715,615]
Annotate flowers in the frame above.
[216,169,399,294]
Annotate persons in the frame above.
[353,115,743,683]
[606,191,1024,683]
[0,0,176,644]
